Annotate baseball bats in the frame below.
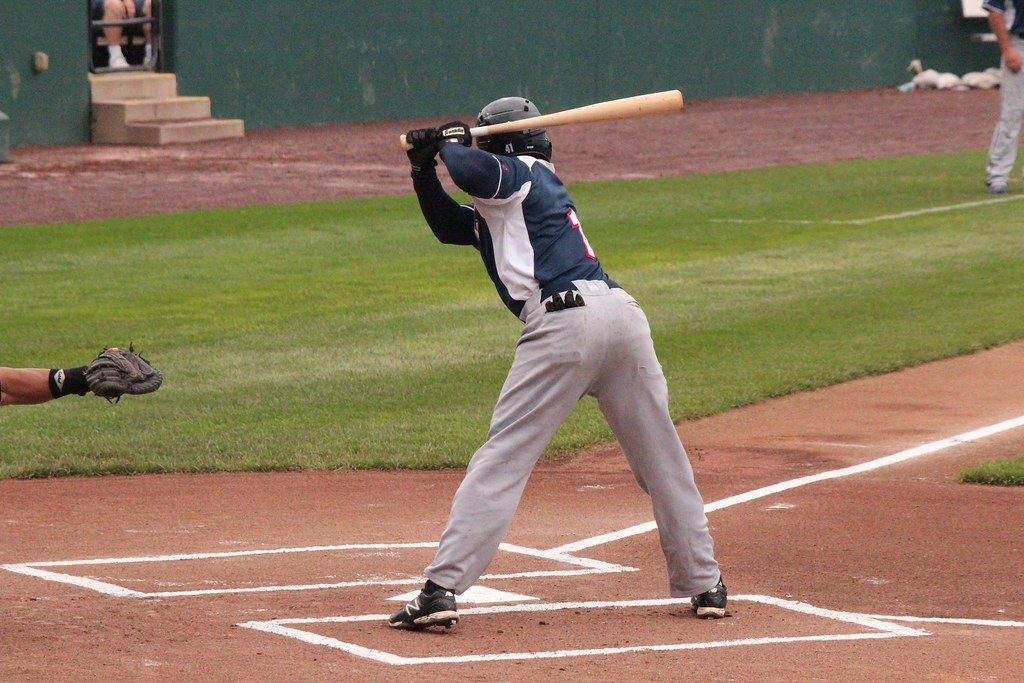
[401,90,684,152]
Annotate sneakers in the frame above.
[691,584,727,619]
[388,589,460,629]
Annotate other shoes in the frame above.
[108,45,130,70]
[144,44,152,67]
[990,182,1007,194]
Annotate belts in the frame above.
[540,280,623,305]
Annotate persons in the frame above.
[92,0,161,68]
[0,342,163,406]
[982,0,1024,193]
[386,97,726,631]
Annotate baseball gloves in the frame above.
[83,333,162,403]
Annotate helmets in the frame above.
[474,97,552,163]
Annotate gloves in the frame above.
[438,121,472,151]
[545,290,585,314]
[406,127,438,183]
